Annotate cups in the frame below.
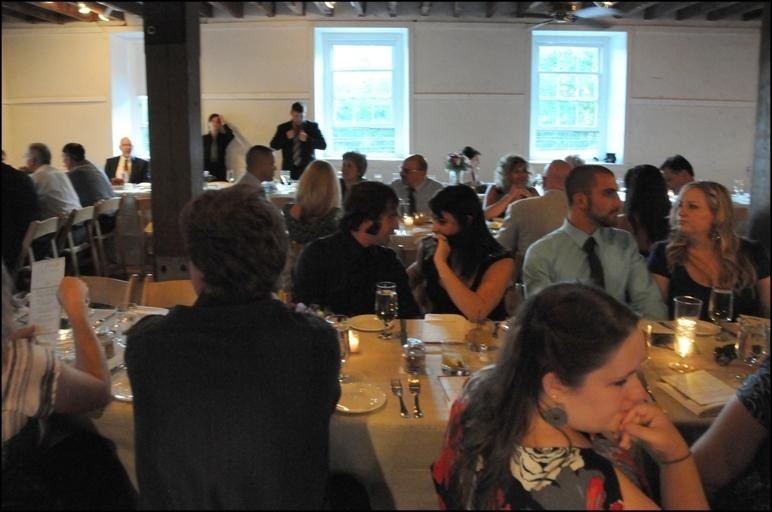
[111,308,148,400]
[226,170,235,186]
[502,283,528,330]
[708,287,734,341]
[667,296,703,373]
[735,316,769,382]
[375,282,397,339]
[280,171,291,186]
[325,315,352,383]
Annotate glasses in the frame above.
[403,168,422,173]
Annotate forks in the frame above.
[408,374,424,419]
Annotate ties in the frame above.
[124,159,129,174]
[408,186,417,217]
[293,126,302,165]
[583,239,605,288]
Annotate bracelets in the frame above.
[655,447,695,465]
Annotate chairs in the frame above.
[78,275,138,309]
[12,217,59,291]
[142,274,197,310]
[91,198,122,271]
[56,206,101,279]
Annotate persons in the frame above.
[659,155,694,201]
[1,149,39,269]
[122,181,342,512]
[427,282,710,512]
[60,143,118,232]
[337,151,368,203]
[104,139,149,186]
[497,159,571,261]
[271,100,327,181]
[647,181,772,320]
[481,154,540,220]
[17,144,86,247]
[612,166,671,260]
[391,155,443,216]
[451,145,487,191]
[687,348,771,511]
[281,162,341,273]
[293,181,425,321]
[523,165,671,322]
[202,114,235,182]
[227,144,278,205]
[419,183,516,321]
[0,264,136,512]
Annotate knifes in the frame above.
[390,378,410,418]
[399,318,409,345]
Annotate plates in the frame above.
[350,314,395,332]
[670,319,722,337]
[99,339,125,372]
[335,382,388,413]
